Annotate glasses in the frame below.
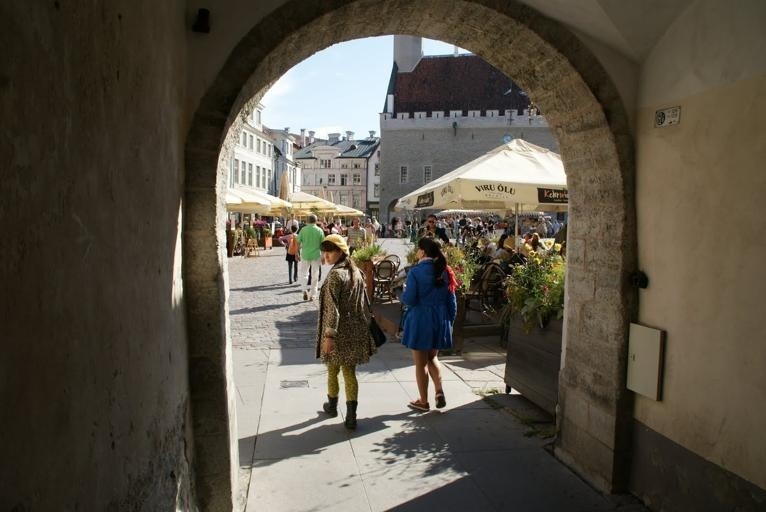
[428,220,435,223]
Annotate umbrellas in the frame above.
[279,171,292,201]
[394,138,570,254]
[226,182,367,247]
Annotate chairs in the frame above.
[372,254,400,306]
[466,262,506,312]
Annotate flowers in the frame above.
[503,239,566,335]
[406,234,473,294]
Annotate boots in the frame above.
[323,394,338,417]
[346,400,358,429]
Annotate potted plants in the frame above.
[350,238,387,301]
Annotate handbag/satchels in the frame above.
[367,318,387,348]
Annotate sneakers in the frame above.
[435,391,446,408]
[289,277,298,284]
[410,400,430,411]
[302,290,316,301]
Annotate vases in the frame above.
[454,288,468,318]
[504,309,563,425]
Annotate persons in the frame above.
[278,225,301,285]
[296,214,325,301]
[314,233,378,430]
[226,214,384,255]
[388,215,567,257]
[399,237,458,412]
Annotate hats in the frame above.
[537,216,545,221]
[321,234,350,255]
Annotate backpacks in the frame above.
[287,234,299,255]
